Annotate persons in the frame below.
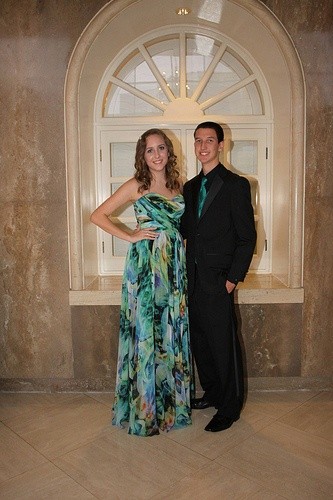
[178,122,257,433]
[90,129,196,437]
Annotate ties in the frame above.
[197,177,207,219]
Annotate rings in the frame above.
[147,233,148,236]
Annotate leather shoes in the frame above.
[191,391,214,409]
[204,410,240,432]
